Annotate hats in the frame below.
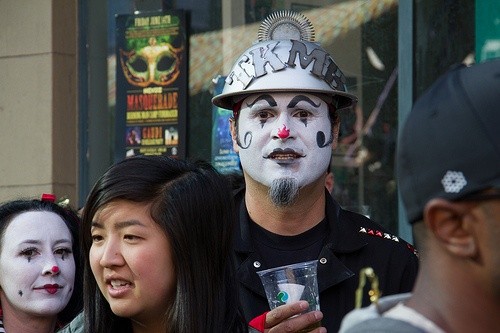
[399,58,500,224]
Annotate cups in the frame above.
[256,260,322,333]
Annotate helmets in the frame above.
[211,9,358,110]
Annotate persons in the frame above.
[0,199,89,333]
[52,155,250,333]
[341,57,500,333]
[211,40,421,333]
